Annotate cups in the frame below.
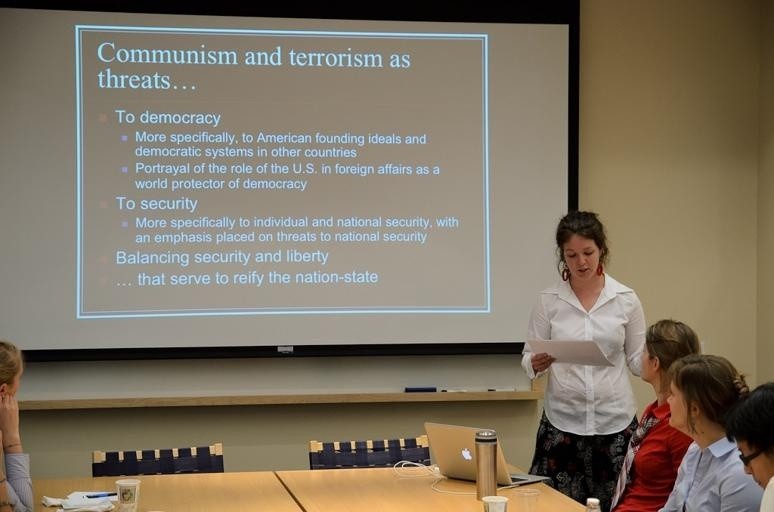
[479,495,508,512]
[115,479,140,512]
[511,487,540,512]
[475,430,498,502]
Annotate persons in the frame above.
[0,430,15,512]
[657,355,764,511]
[522,212,646,512]
[725,382,774,511]
[608,320,700,512]
[0,341,35,512]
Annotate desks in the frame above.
[32,471,306,512]
[274,462,589,512]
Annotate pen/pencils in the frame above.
[87,493,117,498]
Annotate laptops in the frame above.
[425,423,551,490]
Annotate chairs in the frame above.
[308,435,429,469]
[91,442,224,477]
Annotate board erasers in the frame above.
[405,387,437,392]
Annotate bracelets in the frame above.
[0,501,14,507]
[3,442,23,448]
[0,478,5,483]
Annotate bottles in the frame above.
[584,497,601,511]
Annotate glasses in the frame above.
[739,448,768,466]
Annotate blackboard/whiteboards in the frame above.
[0,353,542,411]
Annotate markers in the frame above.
[442,389,467,393]
[488,388,515,392]
